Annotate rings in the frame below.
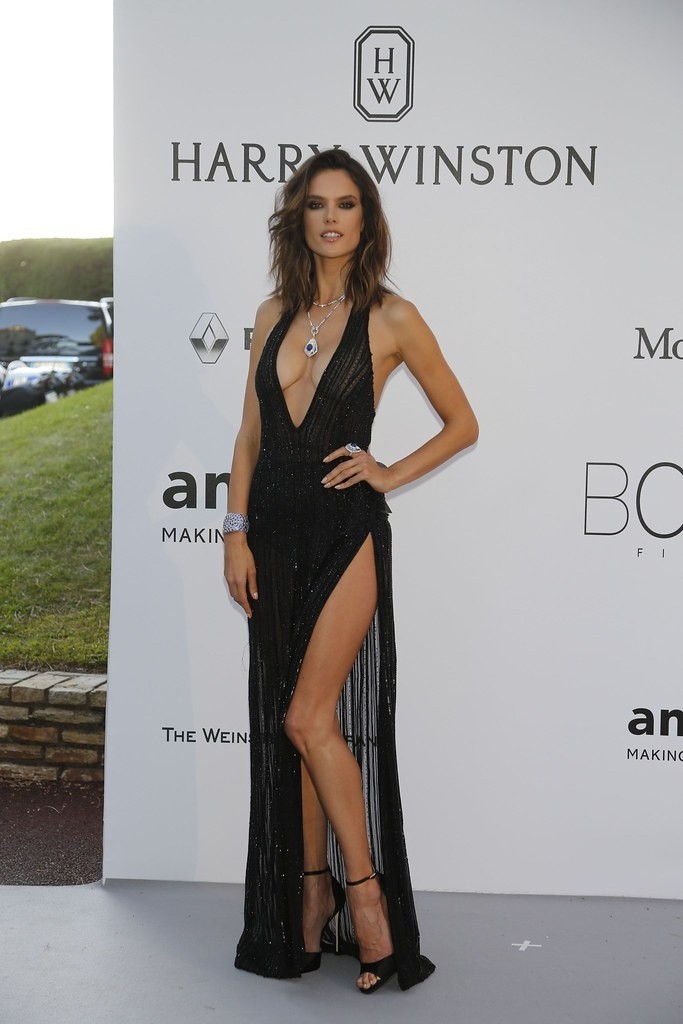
[345,441,361,456]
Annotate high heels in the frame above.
[346,866,396,995]
[302,865,345,973]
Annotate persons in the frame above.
[216,149,479,995]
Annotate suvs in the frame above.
[0,297,113,382]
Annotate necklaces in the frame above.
[304,289,346,358]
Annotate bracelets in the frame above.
[223,513,249,535]
